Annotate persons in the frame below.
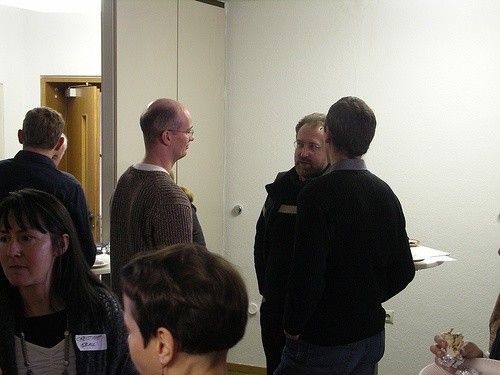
[119,243,249,375]
[273,96,415,375]
[421,293,500,375]
[0,98,208,375]
[254,112,330,375]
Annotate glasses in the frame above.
[160,129,194,138]
[294,140,321,152]
